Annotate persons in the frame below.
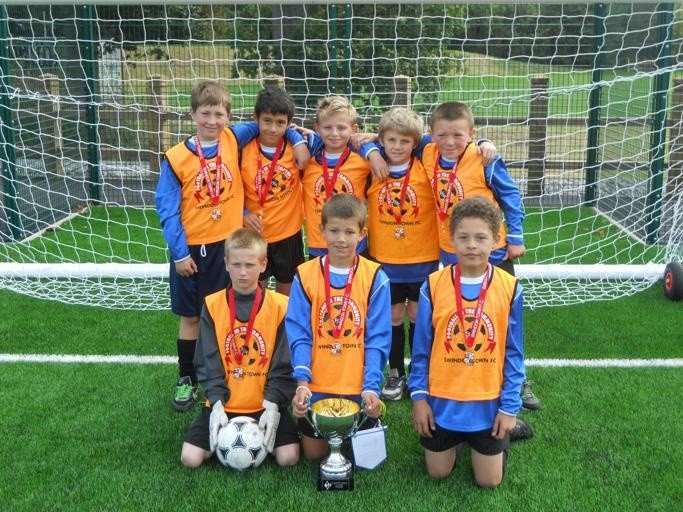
[236,83,390,296]
[301,95,389,263]
[284,193,386,462]
[179,227,301,468]
[289,107,498,403]
[407,195,533,488]
[153,81,310,412]
[349,101,540,411]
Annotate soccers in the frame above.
[215,416,268,474]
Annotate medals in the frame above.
[330,342,342,356]
[463,352,477,365]
[394,224,406,240]
[234,367,244,380]
[211,207,221,221]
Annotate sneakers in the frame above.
[171,376,201,411]
[522,381,539,409]
[381,368,407,401]
[509,418,534,441]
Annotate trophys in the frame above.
[301,395,371,492]
